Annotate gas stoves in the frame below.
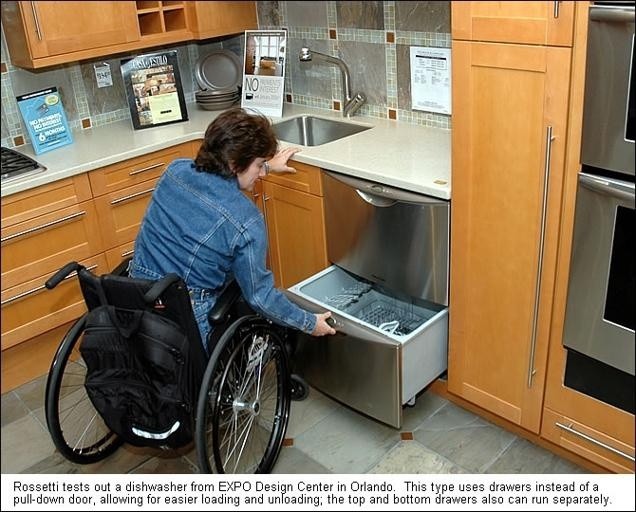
[0,146,47,182]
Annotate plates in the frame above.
[195,48,243,94]
[195,89,241,111]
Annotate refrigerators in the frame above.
[282,169,453,430]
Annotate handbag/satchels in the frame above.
[78,305,192,449]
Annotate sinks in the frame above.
[271,115,377,147]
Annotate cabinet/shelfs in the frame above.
[1,0,258,74]
[0,139,331,396]
[448,1,575,436]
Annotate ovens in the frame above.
[560,0,636,377]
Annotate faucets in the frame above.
[299,47,367,117]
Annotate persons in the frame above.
[122,106,336,358]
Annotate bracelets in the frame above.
[264,162,269,175]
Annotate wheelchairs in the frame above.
[43,257,310,475]
